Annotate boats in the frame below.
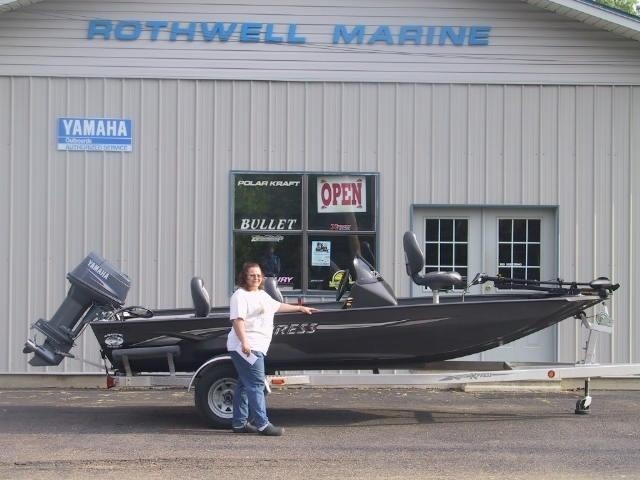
[20,231,620,428]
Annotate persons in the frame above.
[226,263,320,436]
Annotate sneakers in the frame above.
[256,424,285,436]
[233,422,257,433]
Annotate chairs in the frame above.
[401,231,462,304]
[190,276,213,319]
